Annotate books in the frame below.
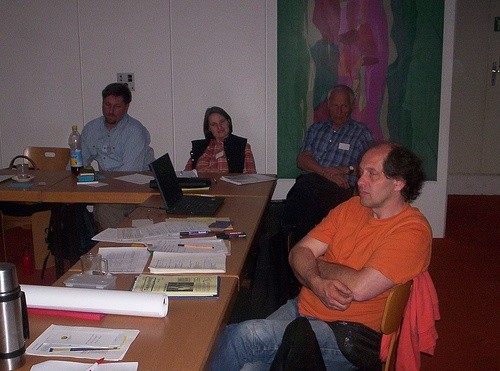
[165,217,233,230]
[219,174,277,185]
[24,323,141,361]
[0,176,13,184]
[91,219,231,256]
[26,307,106,320]
[129,274,221,299]
[29,360,139,371]
[177,177,211,190]
[149,250,227,274]
[115,173,155,185]
[90,246,150,274]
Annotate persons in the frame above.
[283,83,373,248]
[65,83,157,235]
[185,106,258,174]
[209,142,433,371]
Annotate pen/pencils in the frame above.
[86,357,105,371]
[178,244,214,248]
[217,231,246,238]
[180,234,215,239]
[180,170,183,175]
[213,177,218,182]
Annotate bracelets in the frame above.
[348,165,354,175]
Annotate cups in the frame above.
[80,254,108,275]
[16,164,29,179]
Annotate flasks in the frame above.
[0,262,30,371]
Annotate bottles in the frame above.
[68,125,82,178]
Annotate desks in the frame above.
[0,170,278,371]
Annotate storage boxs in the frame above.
[31,210,56,270]
[63,273,117,290]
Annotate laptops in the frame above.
[150,152,226,216]
[150,177,211,189]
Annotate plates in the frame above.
[11,176,35,183]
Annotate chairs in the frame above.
[381,278,413,371]
[23,147,71,170]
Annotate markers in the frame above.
[180,231,208,235]
